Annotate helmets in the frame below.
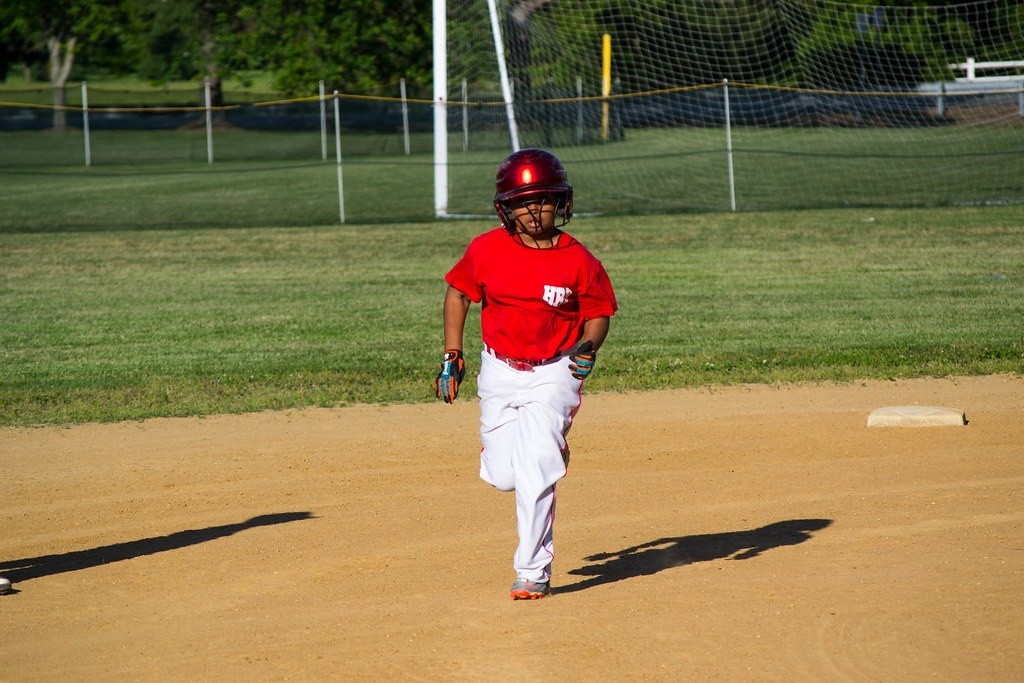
[493,149,573,232]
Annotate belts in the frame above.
[487,346,562,371]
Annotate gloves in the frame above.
[434,349,465,404]
[568,340,596,380]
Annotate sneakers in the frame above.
[510,576,550,599]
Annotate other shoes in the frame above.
[0,577,11,594]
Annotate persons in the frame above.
[436,149,618,599]
